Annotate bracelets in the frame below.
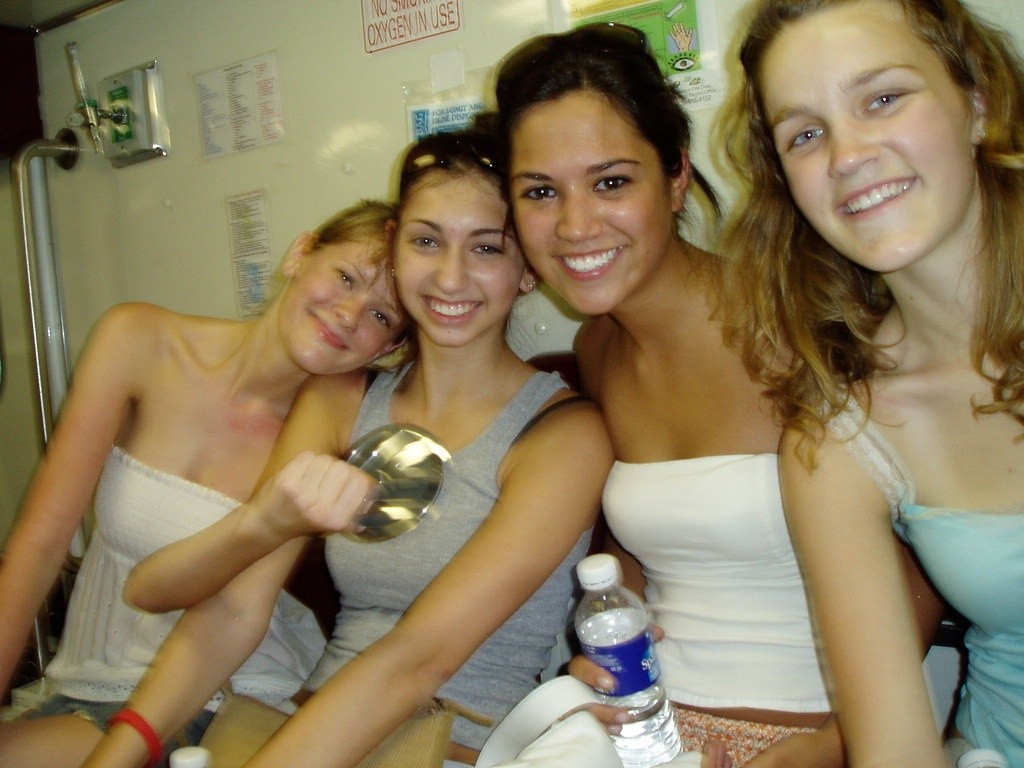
[105,708,162,768]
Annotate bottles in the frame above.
[170,748,212,768]
[957,750,1010,768]
[572,554,682,768]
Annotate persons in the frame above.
[79,112,612,768]
[707,0,1024,768]
[496,20,944,766]
[0,196,416,768]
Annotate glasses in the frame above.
[402,152,504,188]
[495,22,655,85]
